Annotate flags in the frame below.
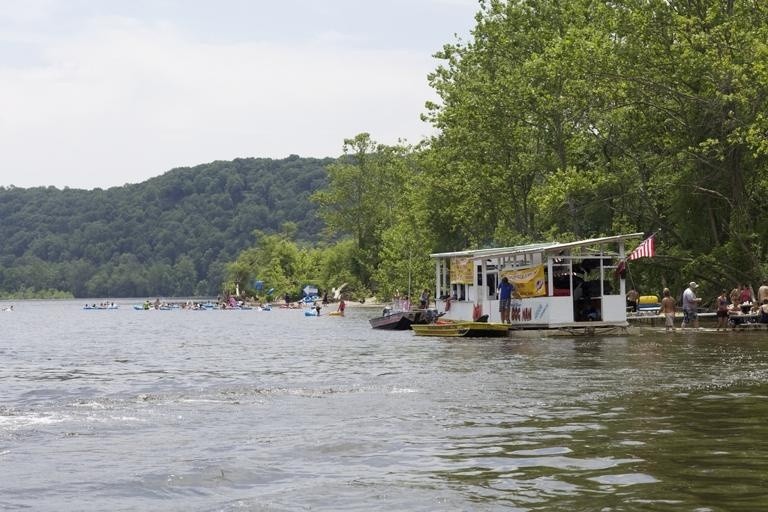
[628,235,655,261]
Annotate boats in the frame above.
[368,298,514,339]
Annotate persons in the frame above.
[393,288,410,311]
[143,293,290,310]
[715,280,768,328]
[498,277,515,324]
[680,282,702,329]
[419,289,431,309]
[657,288,677,331]
[313,290,346,315]
[85,301,114,307]
[626,287,640,312]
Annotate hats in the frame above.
[690,281,699,289]
